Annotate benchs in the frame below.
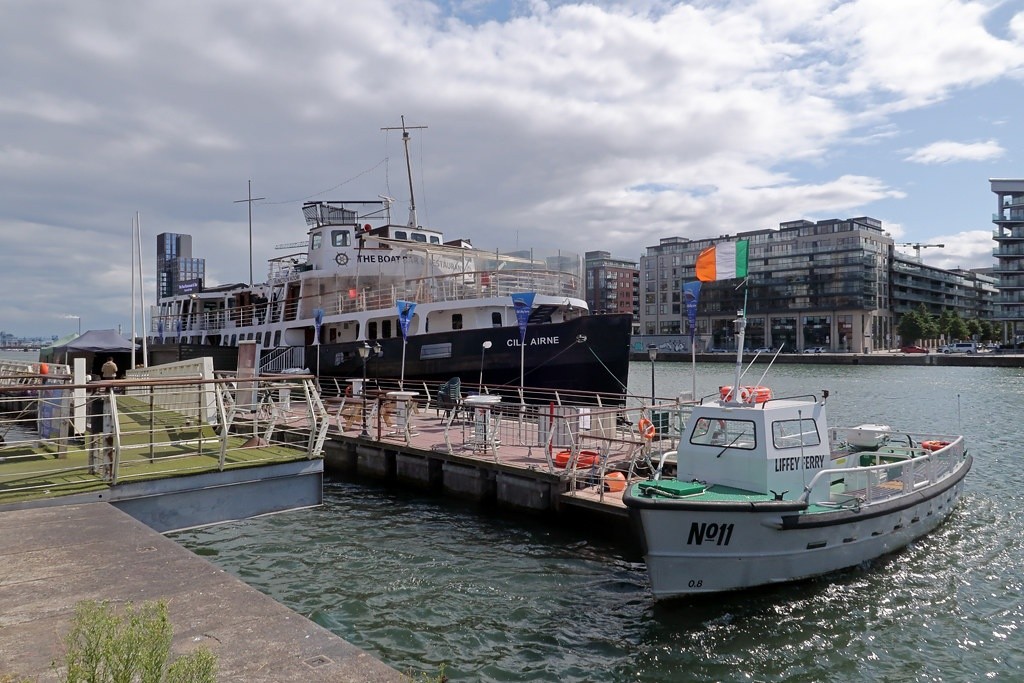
[308,402,419,418]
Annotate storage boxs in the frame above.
[847,424,891,447]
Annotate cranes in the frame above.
[894,242,945,263]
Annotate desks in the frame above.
[366,389,419,425]
[316,398,374,430]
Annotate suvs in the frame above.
[942,342,975,355]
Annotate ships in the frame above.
[127,108,637,423]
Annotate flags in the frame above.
[695,240,748,282]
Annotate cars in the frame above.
[900,345,929,353]
[803,347,827,353]
[937,345,947,353]
[753,347,771,353]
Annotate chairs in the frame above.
[436,377,462,426]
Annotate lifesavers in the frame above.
[922,440,952,451]
[720,385,770,404]
[346,385,352,397]
[639,418,656,438]
[555,453,599,469]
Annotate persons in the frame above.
[101,357,118,392]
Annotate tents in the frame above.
[40,329,142,367]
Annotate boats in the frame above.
[620,236,974,605]
